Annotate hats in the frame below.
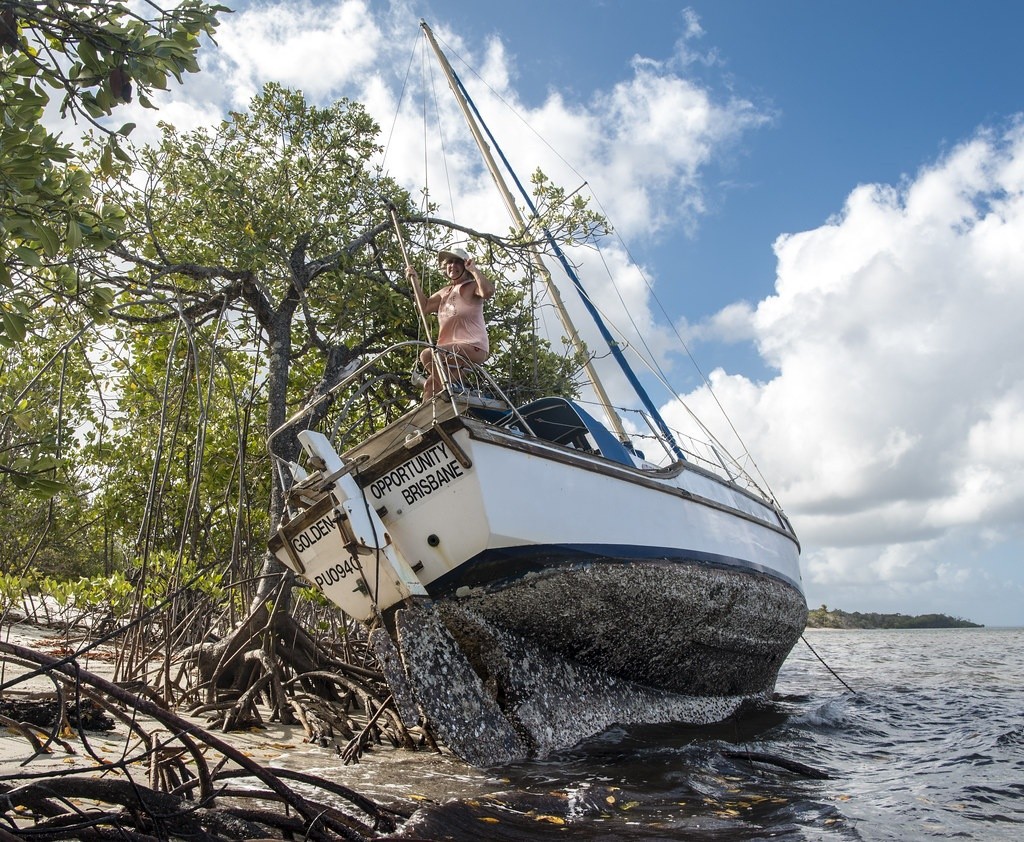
[438,248,469,267]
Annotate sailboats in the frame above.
[266,19,809,770]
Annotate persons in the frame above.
[405,247,496,404]
